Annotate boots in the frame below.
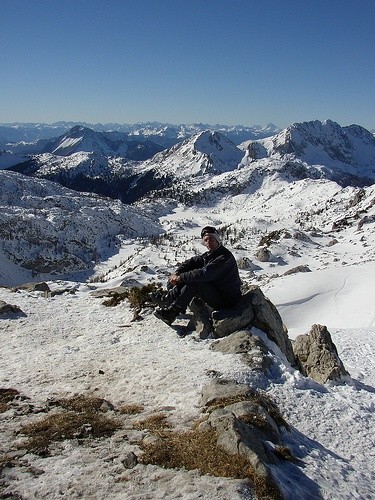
[151,291,169,308]
[154,302,181,325]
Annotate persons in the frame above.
[152,226,241,326]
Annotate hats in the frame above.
[201,226,220,244]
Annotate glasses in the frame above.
[201,227,215,239]
